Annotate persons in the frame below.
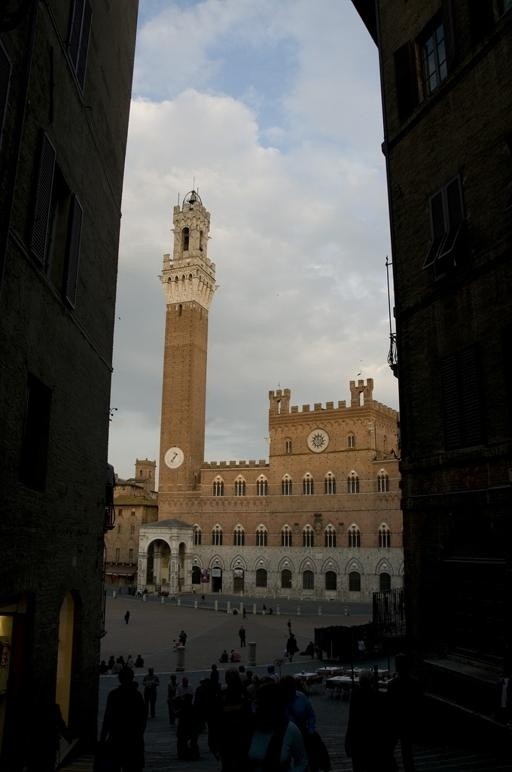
[99,588,420,771]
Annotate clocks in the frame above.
[164,448,184,469]
[306,428,330,454]
